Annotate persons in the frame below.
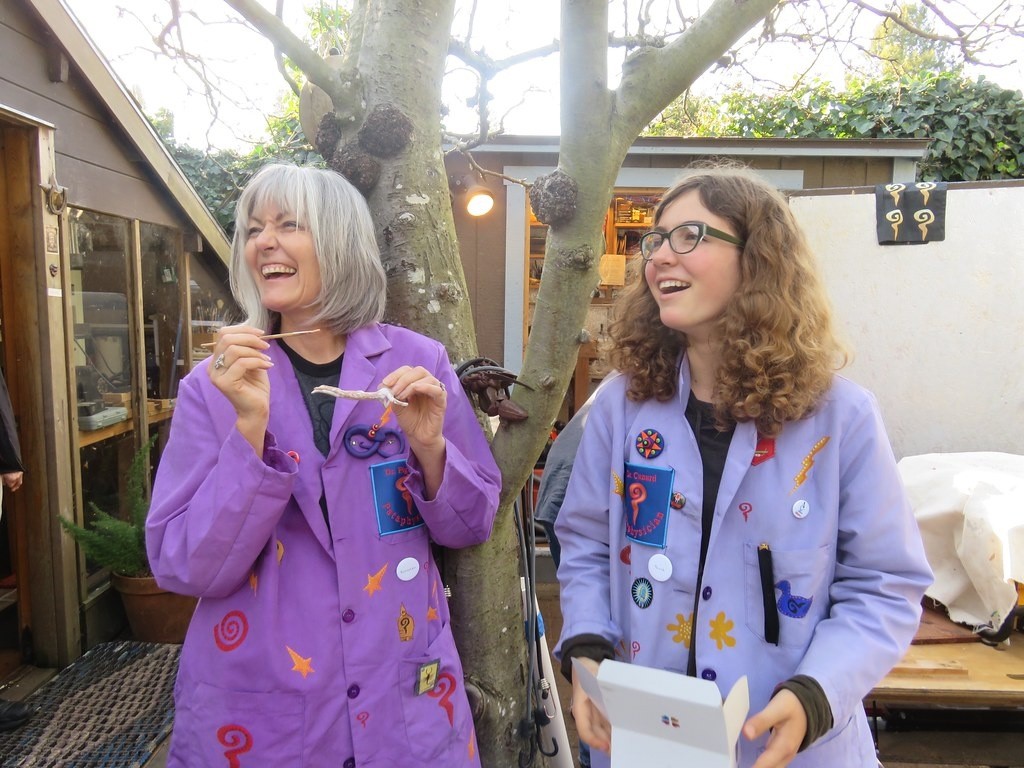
[0,320,34,734]
[558,175,935,768]
[141,163,503,768]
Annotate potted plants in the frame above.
[56,429,199,644]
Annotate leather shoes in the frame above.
[0,698,32,728]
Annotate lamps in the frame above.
[448,170,496,217]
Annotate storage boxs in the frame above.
[570,657,751,768]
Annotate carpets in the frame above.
[0,646,179,768]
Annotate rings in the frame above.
[214,354,227,371]
[440,382,446,392]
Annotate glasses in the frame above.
[640,221,747,261]
